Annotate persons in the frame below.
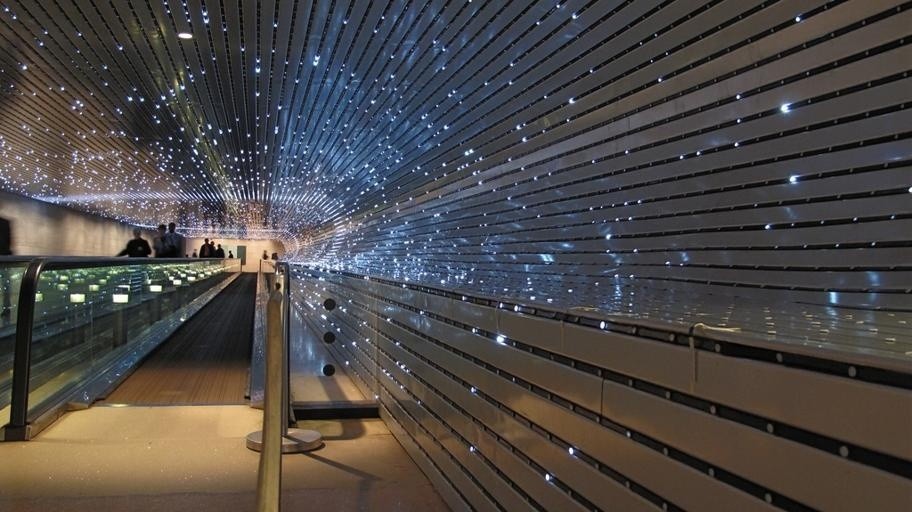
[152,222,165,257]
[228,250,234,259]
[190,248,198,257]
[262,249,268,259]
[200,238,225,257]
[163,223,188,257]
[115,227,152,258]
